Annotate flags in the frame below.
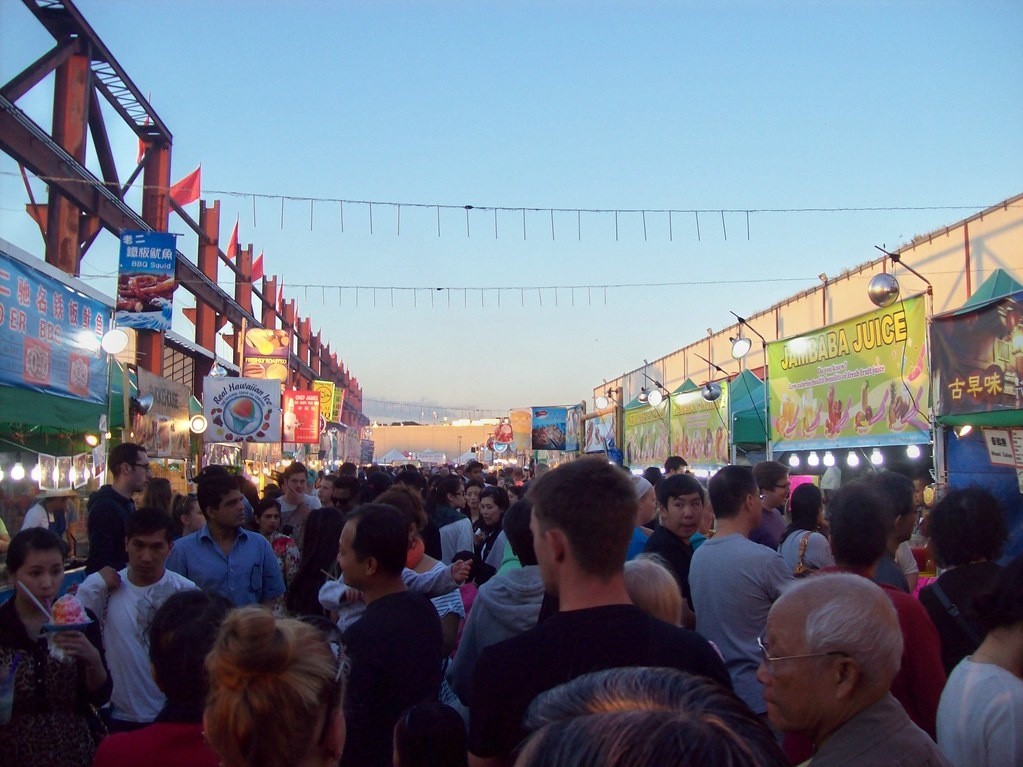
[252,253,263,283]
[225,215,239,266]
[169,167,201,213]
[137,116,150,164]
[278,281,283,315]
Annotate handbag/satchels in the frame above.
[793,531,816,577]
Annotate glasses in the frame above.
[452,492,466,496]
[751,494,766,504]
[757,637,852,674]
[775,482,791,490]
[330,494,354,505]
[130,463,150,472]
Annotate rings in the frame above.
[72,643,76,649]
[78,631,81,637]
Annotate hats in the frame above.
[188,465,230,484]
[465,461,484,473]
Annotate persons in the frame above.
[0,443,1023,767]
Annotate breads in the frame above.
[243,328,289,380]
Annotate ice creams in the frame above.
[49,593,90,625]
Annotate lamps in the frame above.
[101,329,128,355]
[595,396,608,409]
[701,383,722,402]
[729,337,751,358]
[189,415,207,433]
[648,390,662,407]
[131,392,154,415]
[952,424,971,437]
[868,273,900,307]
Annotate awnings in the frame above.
[953,268,1023,316]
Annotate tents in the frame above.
[731,398,770,466]
